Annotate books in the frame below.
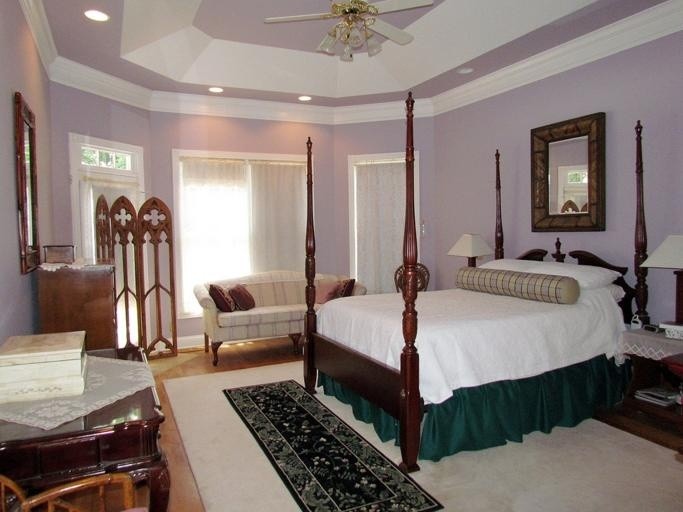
[0,331,89,403]
[658,319,683,331]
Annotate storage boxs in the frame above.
[0,330,86,405]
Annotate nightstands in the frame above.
[622,330,683,437]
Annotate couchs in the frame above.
[193,270,367,367]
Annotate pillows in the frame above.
[228,284,255,311]
[315,283,340,304]
[454,266,580,304]
[477,259,622,290]
[208,284,235,312]
[335,279,355,297]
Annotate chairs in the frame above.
[393,263,430,292]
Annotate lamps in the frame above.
[638,235,683,340]
[313,0,383,62]
[447,233,495,267]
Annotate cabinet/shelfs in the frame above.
[37,259,116,356]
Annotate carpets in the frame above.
[161,360,683,512]
[221,378,446,512]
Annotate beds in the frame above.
[304,90,650,475]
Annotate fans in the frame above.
[263,0,434,62]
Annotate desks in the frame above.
[0,346,171,511]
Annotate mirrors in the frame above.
[14,91,41,275]
[529,112,606,232]
